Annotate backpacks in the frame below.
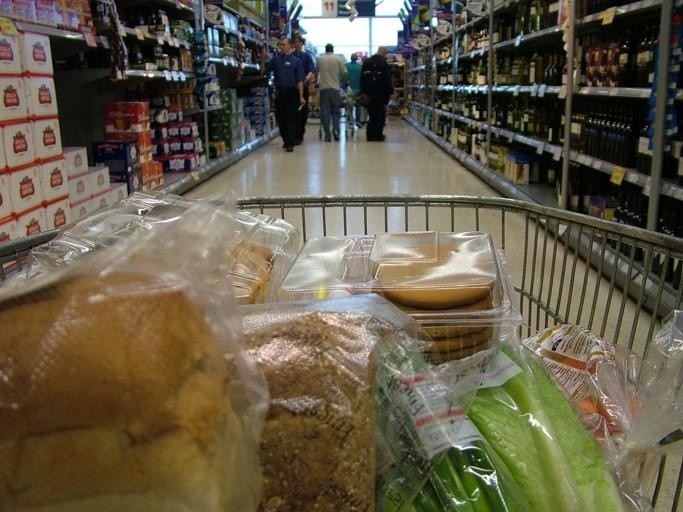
[361,57,381,96]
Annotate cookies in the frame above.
[374,257,497,364]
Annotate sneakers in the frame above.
[366,134,385,141]
[332,130,341,141]
[283,139,293,151]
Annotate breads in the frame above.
[0,274,256,510]
[234,305,382,510]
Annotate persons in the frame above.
[306,39,350,144]
[343,48,368,130]
[359,46,394,144]
[259,37,306,154]
[288,35,315,137]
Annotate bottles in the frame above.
[431,0,683,285]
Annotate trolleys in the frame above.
[0,193,682,512]
[315,81,355,140]
